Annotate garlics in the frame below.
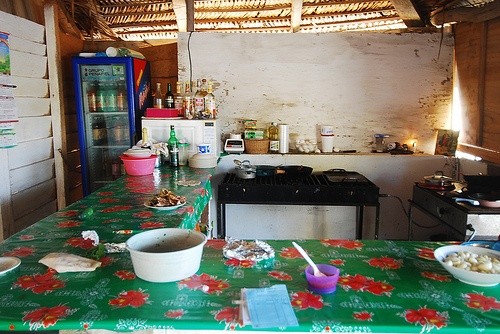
[443,250,500,274]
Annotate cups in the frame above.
[305,264,339,294]
[197,144,210,153]
[376,138,385,152]
[321,126,334,152]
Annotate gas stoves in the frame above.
[412,181,500,235]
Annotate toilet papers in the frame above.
[278,124,290,153]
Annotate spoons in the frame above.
[292,242,327,277]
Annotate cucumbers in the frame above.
[78,208,93,220]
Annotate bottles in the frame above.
[167,124,180,168]
[87,83,131,179]
[106,46,130,57]
[80,207,93,220]
[141,127,152,150]
[154,79,216,119]
[268,121,279,140]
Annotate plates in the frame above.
[143,200,187,210]
[0,256,21,274]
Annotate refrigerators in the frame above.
[72,54,152,198]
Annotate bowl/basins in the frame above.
[434,245,500,287]
[125,228,208,283]
[119,148,158,176]
[189,153,217,169]
[253,165,276,176]
[177,143,191,165]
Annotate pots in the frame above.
[424,170,500,208]
[235,165,257,179]
[275,166,313,178]
